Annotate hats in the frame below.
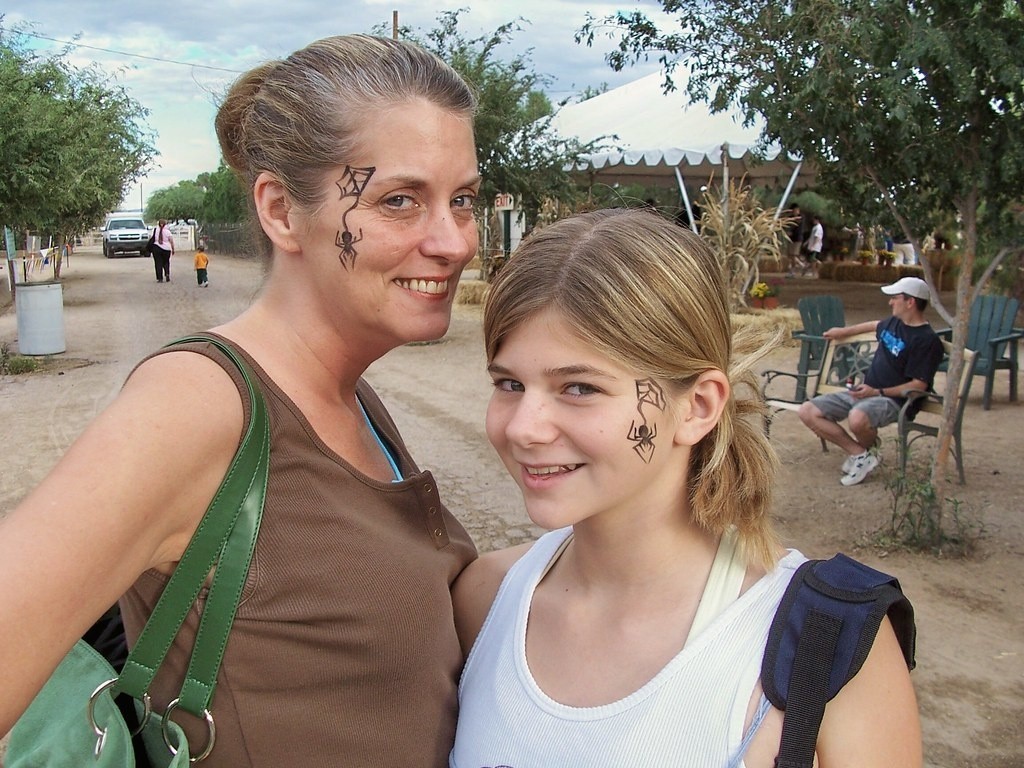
[881,277,930,300]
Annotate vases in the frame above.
[885,259,893,266]
[860,259,869,265]
[878,257,885,265]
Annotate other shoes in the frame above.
[157,279,163,283]
[801,266,808,277]
[785,273,796,279]
[817,262,823,272]
[811,273,819,281]
[197,283,202,287]
[204,281,208,287]
[166,274,170,282]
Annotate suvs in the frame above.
[99,215,152,258]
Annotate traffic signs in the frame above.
[494,192,515,211]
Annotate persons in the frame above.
[446,209,923,768]
[0,35,482,768]
[784,204,952,281]
[151,218,175,282]
[193,245,209,287]
[799,276,945,486]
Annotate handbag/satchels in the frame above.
[0,336,270,768]
[146,228,156,253]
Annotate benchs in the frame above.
[758,334,980,485]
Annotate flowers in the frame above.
[855,250,874,263]
[877,250,888,258]
[886,252,898,261]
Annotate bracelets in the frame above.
[878,387,885,398]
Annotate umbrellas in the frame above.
[479,53,876,293]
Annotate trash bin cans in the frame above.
[14,280,66,355]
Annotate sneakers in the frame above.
[840,450,878,486]
[842,446,882,474]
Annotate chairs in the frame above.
[791,295,874,403]
[935,294,1024,411]
